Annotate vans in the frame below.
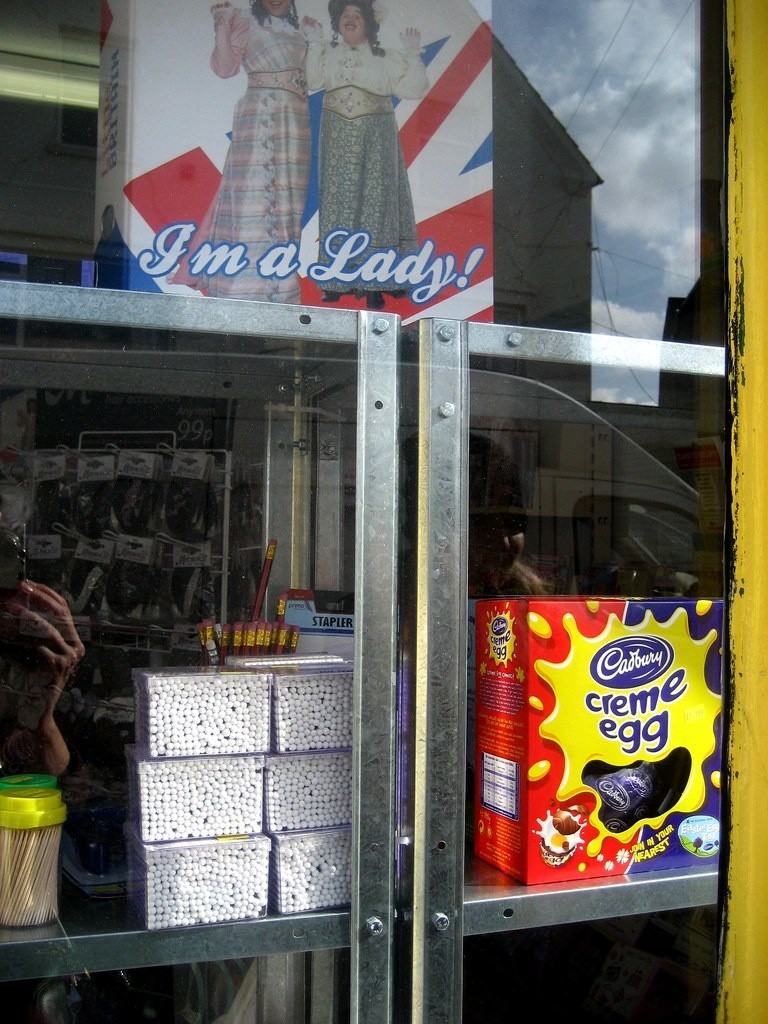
[0,345,705,615]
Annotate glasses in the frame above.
[472,509,530,536]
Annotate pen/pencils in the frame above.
[197,539,300,667]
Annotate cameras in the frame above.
[0,522,27,589]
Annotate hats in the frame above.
[401,432,522,508]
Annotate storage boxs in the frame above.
[473,593,726,892]
[93,0,496,335]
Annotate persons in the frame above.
[94,0,428,310]
[0,580,85,776]
[400,428,550,601]
